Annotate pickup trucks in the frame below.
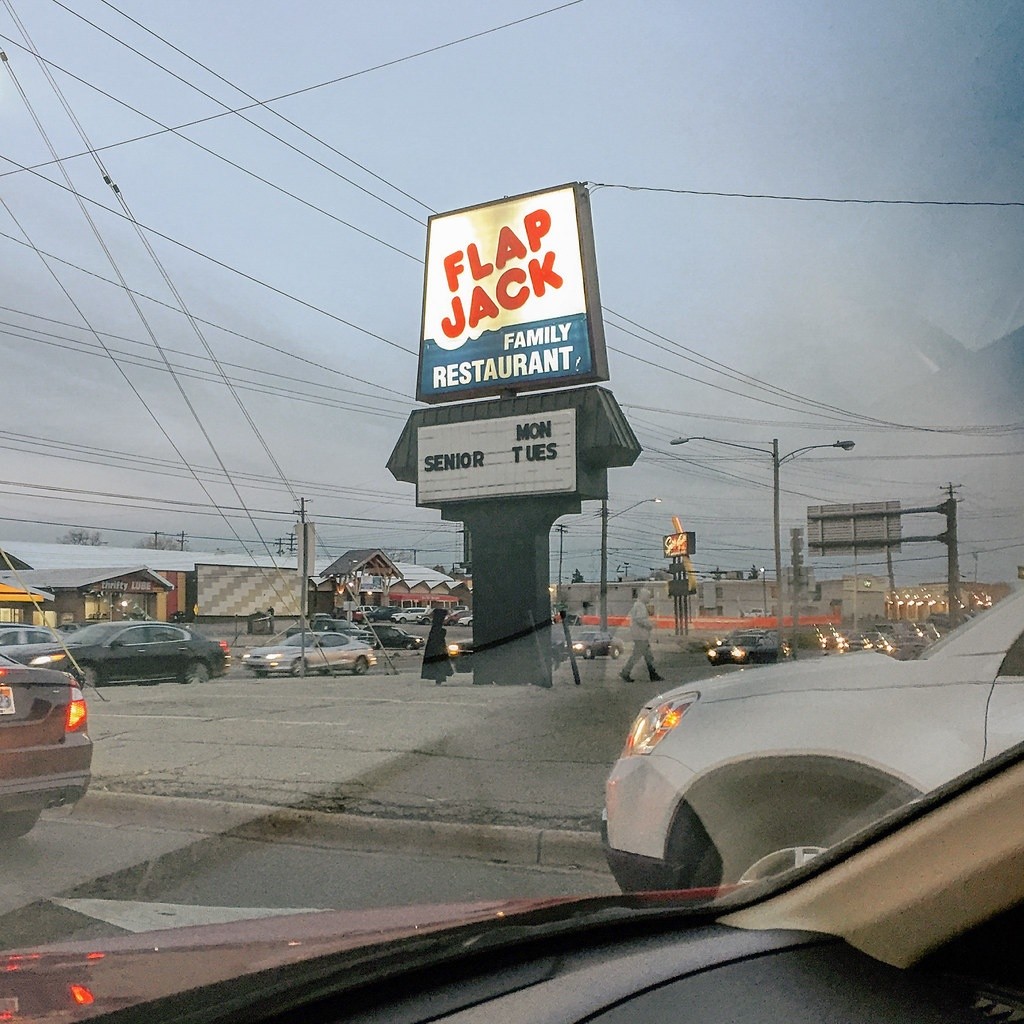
[286,619,377,650]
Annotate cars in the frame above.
[0,621,231,688]
[365,606,403,623]
[241,631,378,678]
[53,622,99,634]
[447,634,566,674]
[786,619,945,661]
[564,631,625,660]
[0,623,37,629]
[332,606,370,624]
[742,608,765,619]
[457,614,473,627]
[443,610,471,626]
[707,629,784,667]
[0,649,94,843]
[415,608,455,625]
[0,627,72,665]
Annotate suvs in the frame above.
[390,607,433,624]
[357,624,426,650]
[599,584,1023,893]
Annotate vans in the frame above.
[309,612,332,630]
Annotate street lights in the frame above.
[669,435,856,627]
[599,498,663,635]
[759,566,767,619]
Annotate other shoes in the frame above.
[617,670,635,683]
[650,672,666,683]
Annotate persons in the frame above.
[617,589,666,682]
[420,609,455,685]
